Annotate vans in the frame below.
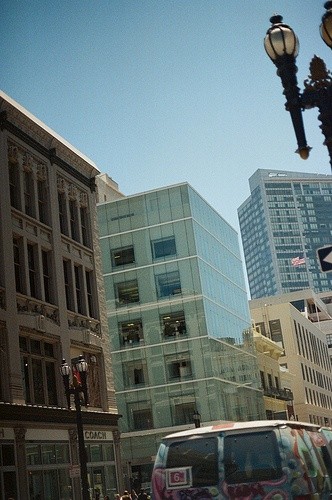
[151,420,332,500]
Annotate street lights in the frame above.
[264,0,332,184]
[60,353,92,500]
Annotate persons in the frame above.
[130,474,142,490]
[95,488,101,500]
[104,495,109,500]
[114,489,150,500]
